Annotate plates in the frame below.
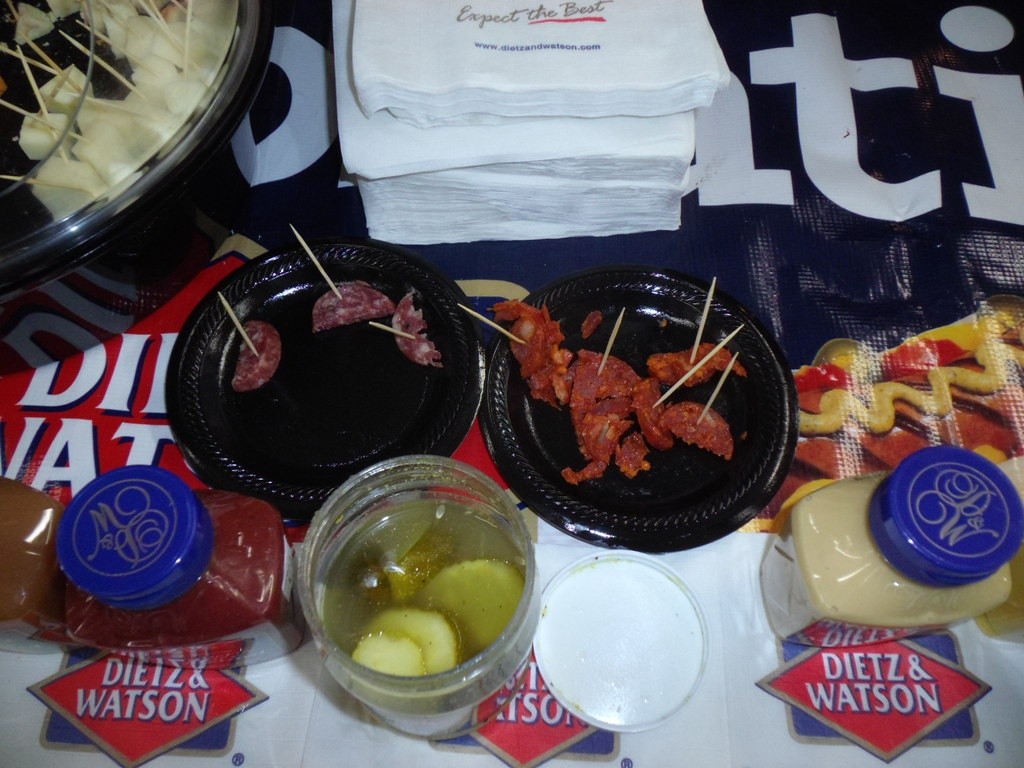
[163,236,485,517]
[0,0,278,302]
[481,266,803,552]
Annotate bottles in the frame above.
[295,455,540,741]
[760,444,1024,649]
[0,464,306,671]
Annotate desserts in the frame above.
[0,0,239,221]
